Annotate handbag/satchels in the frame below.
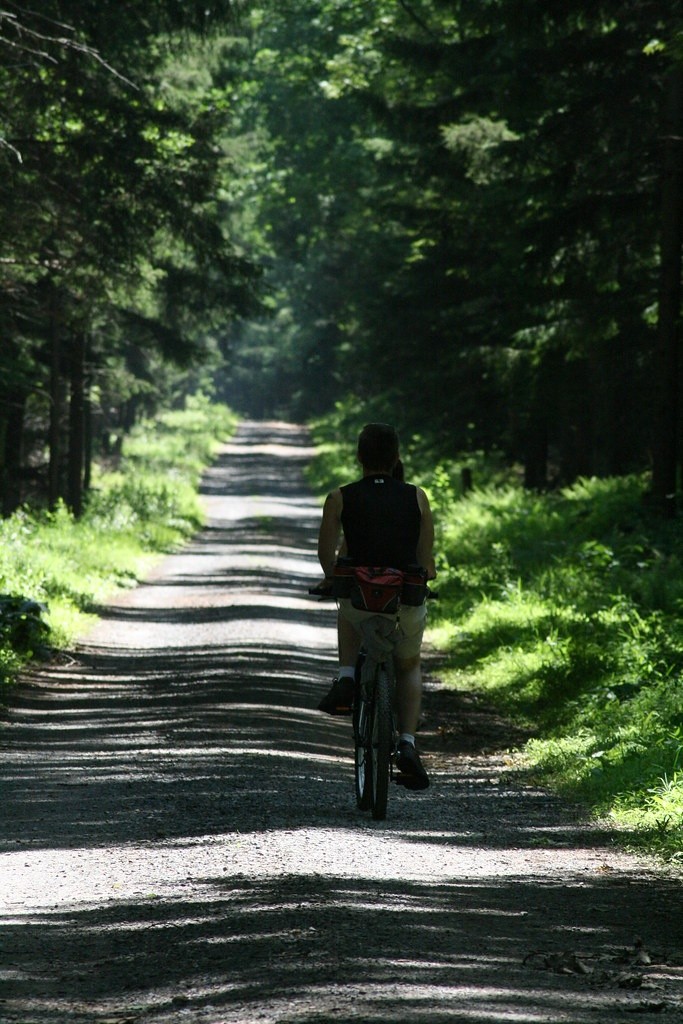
[331,558,429,614]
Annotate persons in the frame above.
[329,456,419,747]
[315,418,440,793]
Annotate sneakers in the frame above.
[396,741,430,790]
[317,677,356,715]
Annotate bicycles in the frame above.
[309,587,440,821]
